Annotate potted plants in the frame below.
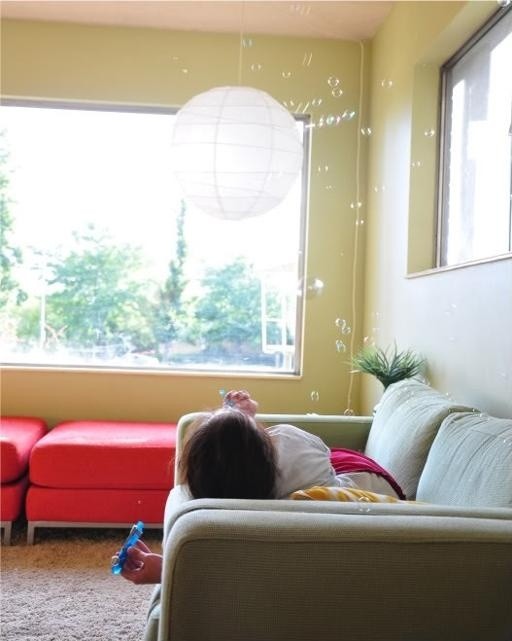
[346,334,431,414]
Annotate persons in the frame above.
[112,387,406,585]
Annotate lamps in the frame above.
[159,1,305,219]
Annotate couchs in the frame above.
[130,375,512,640]
[24,415,178,546]
[0,412,46,551]
